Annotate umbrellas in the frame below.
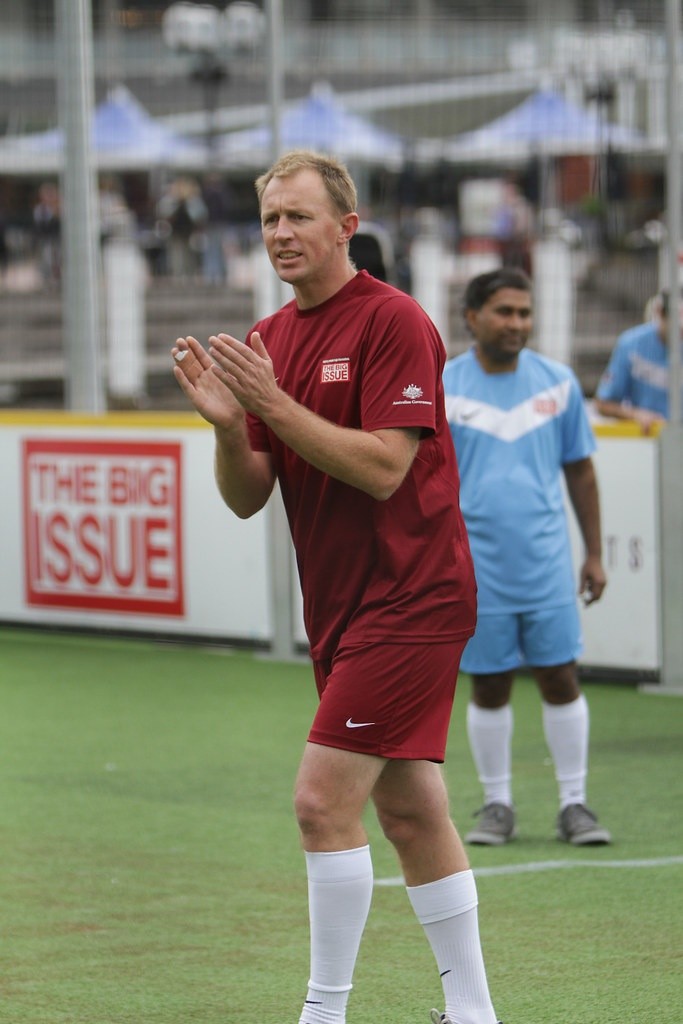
[446,89,671,234]
[0,81,205,179]
[210,79,439,218]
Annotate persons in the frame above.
[592,280,683,437]
[439,266,614,846]
[31,179,210,283]
[171,150,500,1024]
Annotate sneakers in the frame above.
[558,804,610,844]
[465,803,513,845]
